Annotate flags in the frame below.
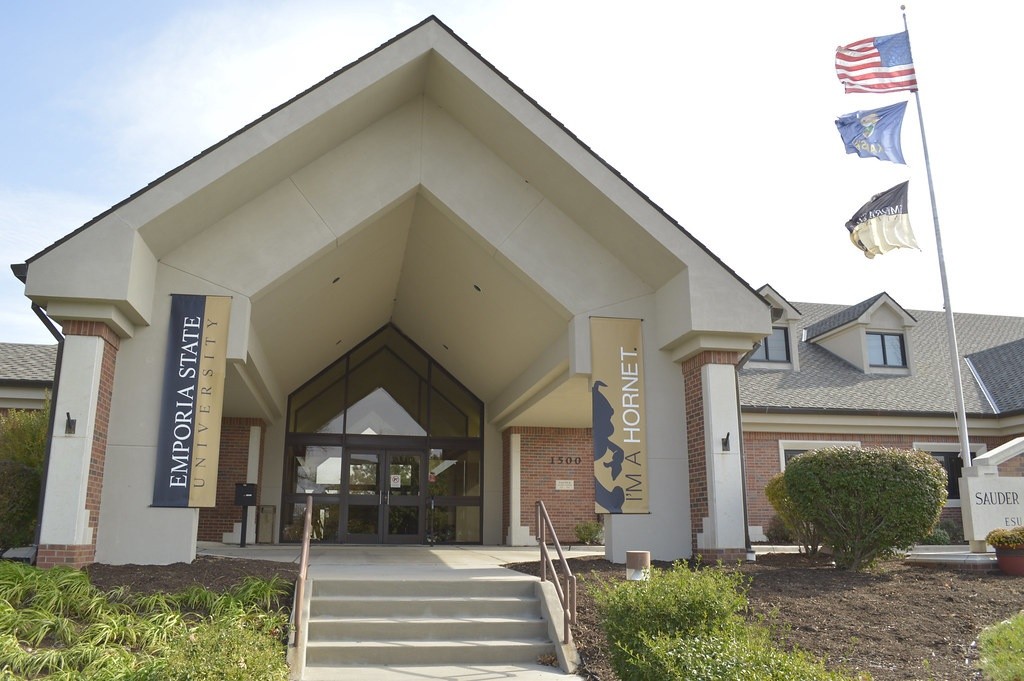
[835,101,908,166]
[845,179,922,259]
[836,29,919,93]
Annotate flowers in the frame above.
[985,524,1024,550]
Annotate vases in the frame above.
[992,543,1024,576]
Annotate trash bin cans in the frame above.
[257,504,276,545]
